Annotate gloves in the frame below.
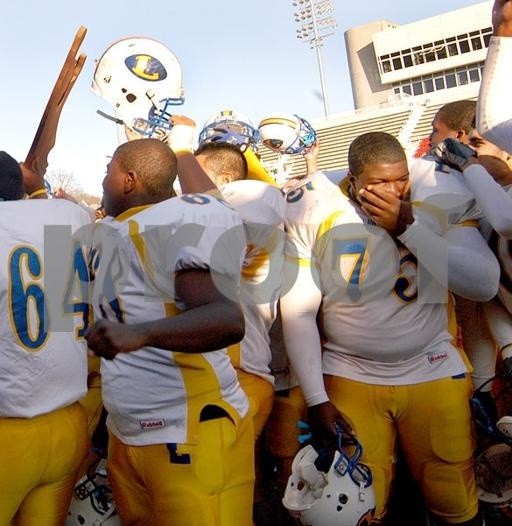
[431,138,479,173]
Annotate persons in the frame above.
[0,149,95,526]
[283,139,321,192]
[82,139,255,525]
[280,133,501,526]
[162,114,275,442]
[429,112,512,418]
[429,100,481,150]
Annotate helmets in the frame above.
[66,460,122,526]
[259,112,317,156]
[280,433,376,525]
[90,35,186,136]
[197,108,262,149]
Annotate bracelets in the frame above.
[28,188,47,198]
